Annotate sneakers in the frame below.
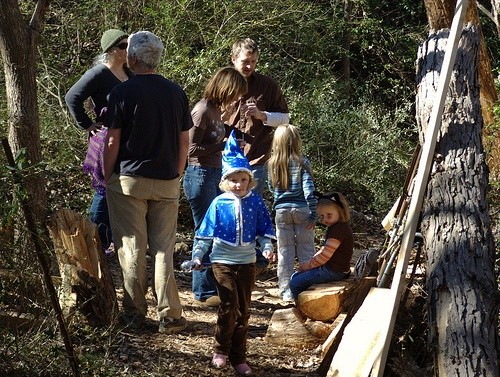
[120,317,147,330]
[159,316,187,335]
[211,352,228,368]
[230,362,252,376]
[283,292,295,302]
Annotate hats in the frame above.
[221,129,254,181]
[100,28,130,54]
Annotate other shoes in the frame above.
[204,295,221,307]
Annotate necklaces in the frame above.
[115,68,125,82]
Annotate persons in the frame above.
[289,191,353,309]
[64,27,317,335]
[190,146,274,377]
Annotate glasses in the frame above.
[113,42,128,50]
[319,214,334,222]
